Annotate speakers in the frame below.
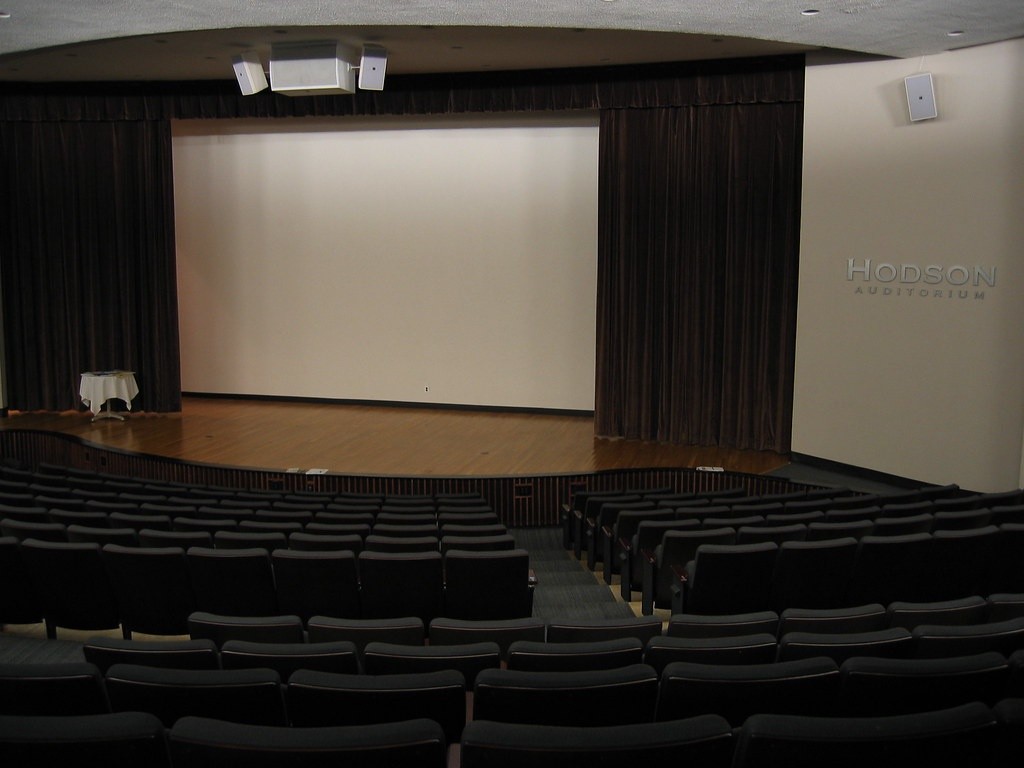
[269,39,355,97]
[358,43,387,91]
[231,51,268,96]
[904,73,938,122]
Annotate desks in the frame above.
[79,370,139,422]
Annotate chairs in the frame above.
[0,456,1024,768]
